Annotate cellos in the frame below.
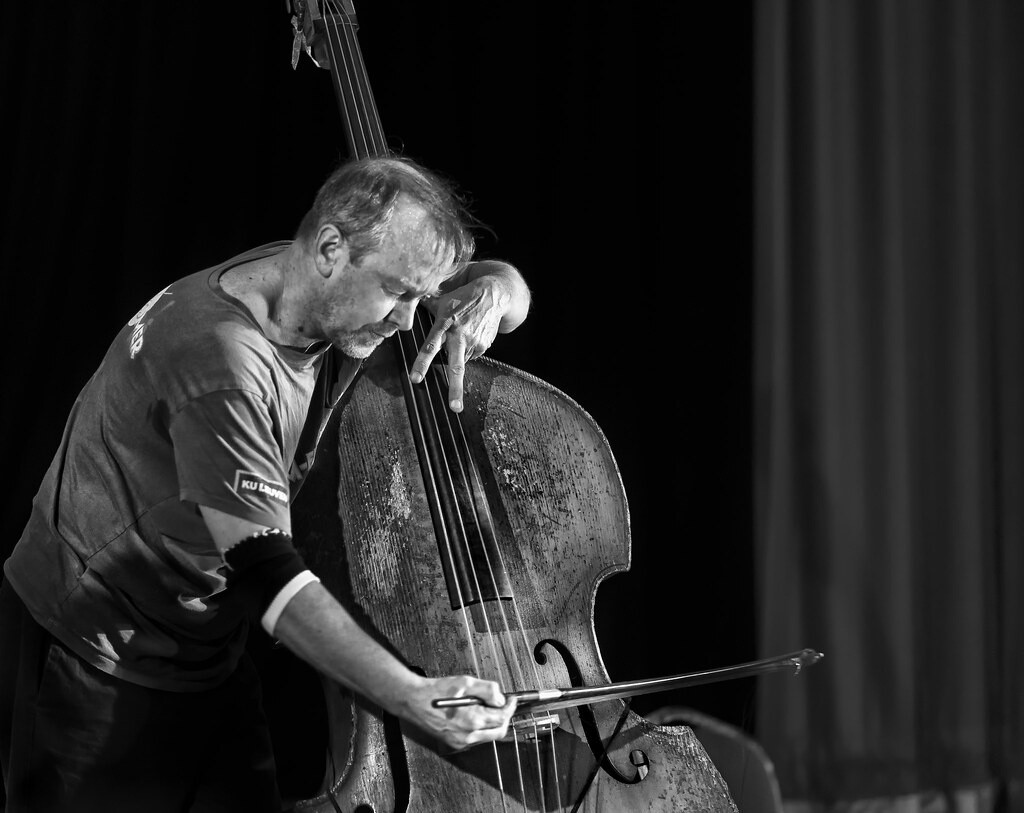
[290,0,742,813]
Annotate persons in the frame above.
[1,159,532,813]
